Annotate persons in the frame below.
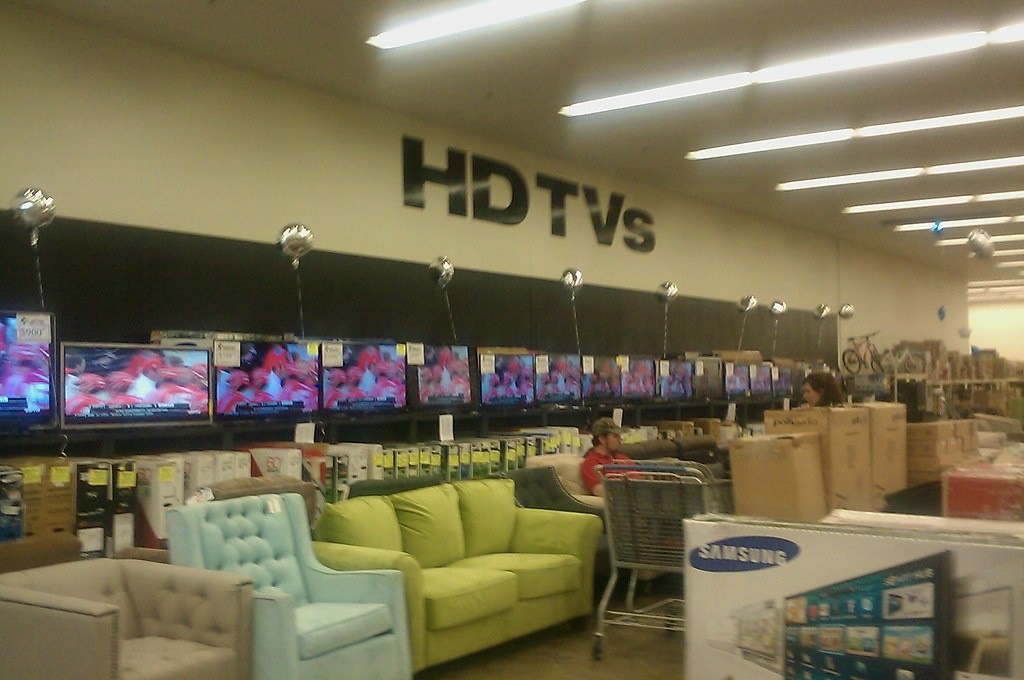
[579,417,646,498]
[0,304,792,417]
[802,371,844,408]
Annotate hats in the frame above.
[590,417,626,438]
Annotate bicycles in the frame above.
[875,340,926,376]
[842,330,884,375]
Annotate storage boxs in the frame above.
[1,442,382,559]
[731,400,907,523]
[893,340,1011,416]
[907,419,979,488]
[648,419,721,444]
[382,427,675,479]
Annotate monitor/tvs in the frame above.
[0,309,793,431]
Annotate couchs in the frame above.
[313,465,612,675]
[164,492,412,680]
[116,476,317,564]
[1,532,256,680]
[528,434,732,595]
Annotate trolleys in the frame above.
[590,458,732,662]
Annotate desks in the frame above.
[942,440,1024,521]
